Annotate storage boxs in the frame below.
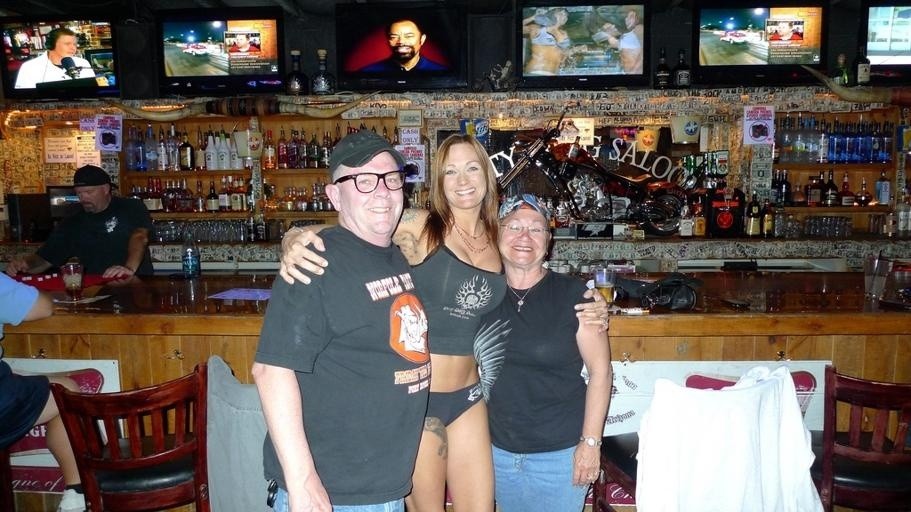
[119,118,252,221]
[256,117,400,227]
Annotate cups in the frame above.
[592,270,618,312]
[56,262,87,315]
[861,254,899,302]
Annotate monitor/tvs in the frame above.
[160,18,280,79]
[0,18,118,99]
[852,0,910,86]
[333,5,467,91]
[691,1,829,89]
[512,0,651,91]
[46,186,81,221]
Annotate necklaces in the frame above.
[455,221,492,254]
[507,273,544,312]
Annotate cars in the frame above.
[185,44,208,56]
[721,30,748,45]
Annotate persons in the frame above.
[599,5,643,74]
[6,165,154,279]
[488,192,613,512]
[229,34,260,53]
[767,22,805,41]
[279,134,610,512]
[251,130,431,512]
[0,271,88,512]
[522,7,570,76]
[14,28,96,89]
[362,17,448,73]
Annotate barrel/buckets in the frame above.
[231,118,265,158]
[669,99,705,144]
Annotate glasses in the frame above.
[500,222,551,235]
[333,170,406,193]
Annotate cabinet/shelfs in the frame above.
[0,269,911,512]
[773,108,902,237]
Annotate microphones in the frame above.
[60,55,77,71]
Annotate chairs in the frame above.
[49,361,213,512]
[808,364,911,512]
[591,369,816,512]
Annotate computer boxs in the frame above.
[8,194,52,242]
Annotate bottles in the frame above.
[855,176,873,206]
[874,166,891,205]
[555,197,570,228]
[326,193,335,211]
[180,127,194,170]
[653,46,670,89]
[228,174,256,211]
[896,187,911,237]
[264,186,281,211]
[677,153,707,190]
[308,176,325,211]
[819,167,839,207]
[897,107,911,152]
[260,129,277,169]
[691,195,707,238]
[167,123,184,171]
[792,213,799,239]
[678,194,692,239]
[333,122,342,150]
[123,125,136,171]
[320,132,329,169]
[784,214,792,239]
[791,181,805,207]
[779,207,785,238]
[760,198,774,239]
[874,216,879,234]
[192,175,207,212]
[567,136,580,159]
[204,125,221,171]
[162,179,194,214]
[878,216,887,234]
[217,125,232,171]
[284,129,301,169]
[218,175,233,212]
[545,197,555,227]
[261,174,270,199]
[231,130,245,171]
[244,129,254,170]
[775,211,781,238]
[885,195,897,239]
[278,126,288,170]
[392,126,399,145]
[671,49,693,89]
[157,126,168,172]
[829,53,853,88]
[284,51,310,95]
[299,129,308,168]
[144,124,157,170]
[195,126,206,172]
[772,113,896,164]
[770,168,780,207]
[327,131,332,168]
[745,191,760,238]
[778,170,791,207]
[381,126,391,145]
[838,171,856,206]
[869,215,874,232]
[703,151,717,188]
[132,176,163,212]
[149,218,284,282]
[804,215,853,238]
[854,45,871,86]
[312,48,335,95]
[206,175,219,213]
[281,186,308,211]
[308,132,320,168]
[135,127,146,170]
[804,175,822,207]
[345,120,377,134]
[716,150,730,188]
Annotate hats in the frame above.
[499,194,551,220]
[329,129,406,173]
[74,164,120,190]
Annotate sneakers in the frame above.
[57,489,86,512]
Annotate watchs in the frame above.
[580,436,603,447]
